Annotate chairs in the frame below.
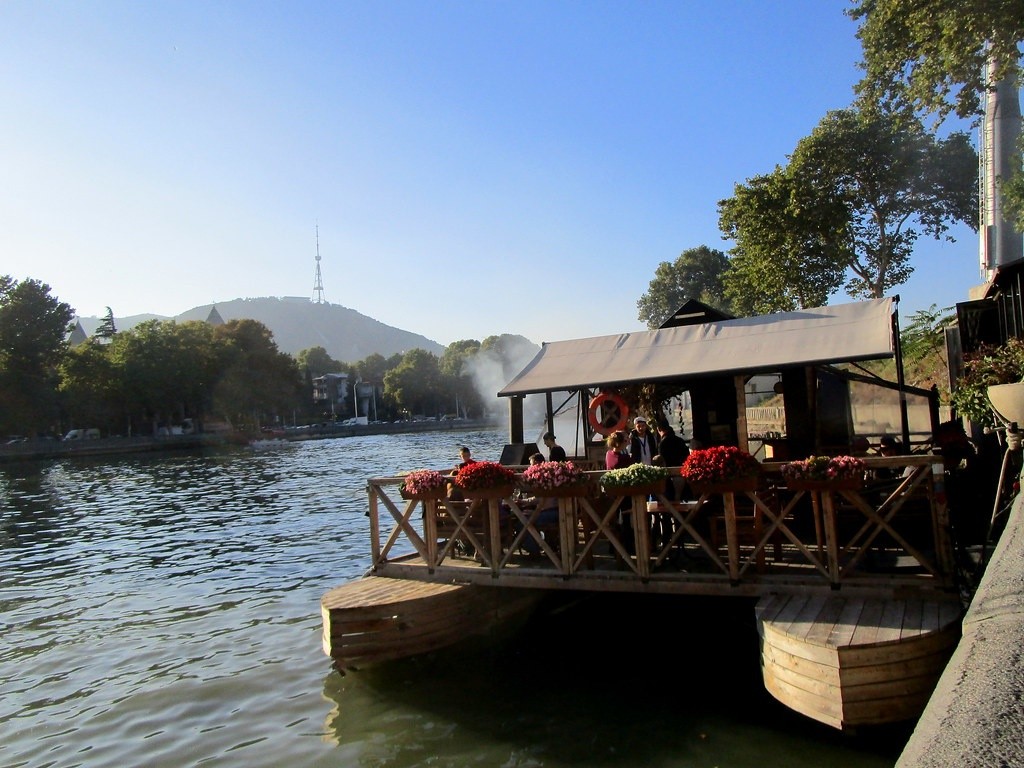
[507,517,560,564]
[709,483,783,573]
[580,495,633,571]
[434,500,513,567]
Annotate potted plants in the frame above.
[947,335,1024,430]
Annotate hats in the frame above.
[634,417,648,425]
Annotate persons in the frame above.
[517,432,566,561]
[458,447,477,471]
[933,421,981,592]
[605,416,690,549]
[875,435,906,478]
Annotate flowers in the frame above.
[679,445,764,487]
[453,461,519,491]
[519,459,591,492]
[598,462,673,490]
[778,454,868,483]
[398,469,443,494]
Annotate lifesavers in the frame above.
[588,392,629,436]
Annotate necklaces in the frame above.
[638,435,646,455]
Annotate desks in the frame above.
[620,500,711,573]
